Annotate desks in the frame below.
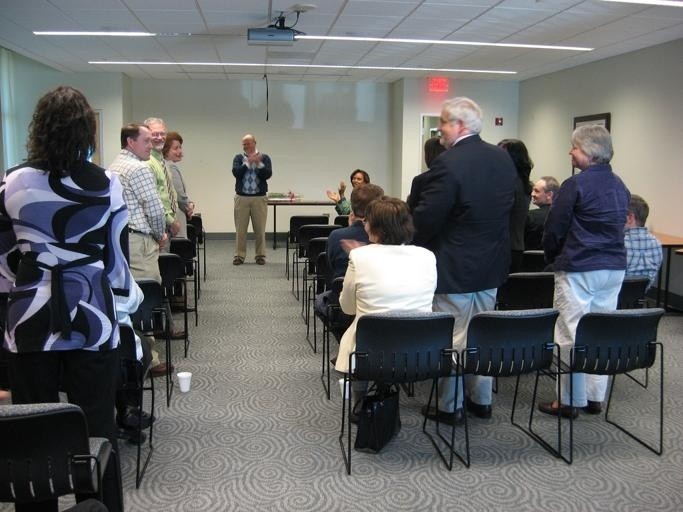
[266,200,338,250]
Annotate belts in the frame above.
[128,228,142,234]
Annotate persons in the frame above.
[406,134,447,216]
[160,131,196,315]
[107,123,176,381]
[110,315,156,445]
[230,134,271,265]
[620,193,662,294]
[326,169,370,215]
[524,174,561,249]
[312,185,382,365]
[0,84,138,512]
[496,139,532,253]
[142,115,190,342]
[414,97,515,424]
[537,122,632,420]
[337,197,438,422]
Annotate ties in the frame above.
[161,159,176,212]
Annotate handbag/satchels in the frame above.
[354,391,401,454]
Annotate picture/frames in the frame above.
[573,111,611,133]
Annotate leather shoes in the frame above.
[466,397,492,419]
[348,412,359,427]
[116,409,156,430]
[122,429,146,445]
[421,405,464,426]
[256,259,265,265]
[233,258,241,265]
[538,402,579,420]
[173,305,195,312]
[580,401,601,414]
[155,331,185,339]
[148,362,174,377]
